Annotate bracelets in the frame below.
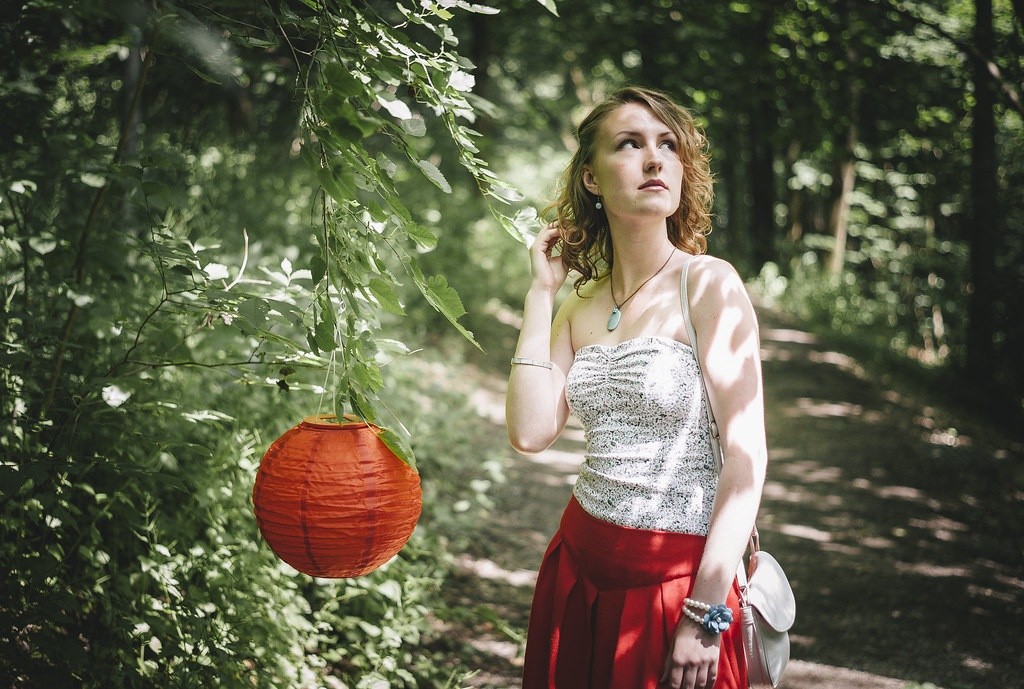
[510,357,554,370]
[682,597,734,635]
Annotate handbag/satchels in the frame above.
[735,550,799,688]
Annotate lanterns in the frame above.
[251,411,424,578]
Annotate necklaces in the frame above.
[607,247,677,332]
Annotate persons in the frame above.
[504,86,769,689]
